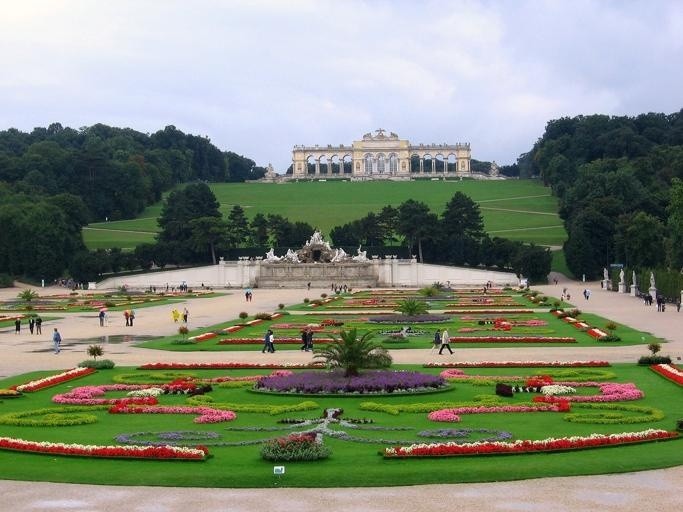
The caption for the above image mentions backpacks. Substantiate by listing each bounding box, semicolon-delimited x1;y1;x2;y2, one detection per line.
56;333;60;341
